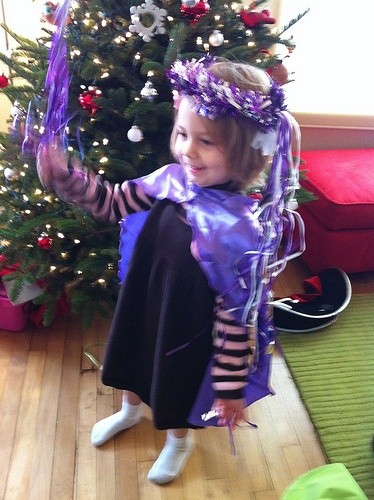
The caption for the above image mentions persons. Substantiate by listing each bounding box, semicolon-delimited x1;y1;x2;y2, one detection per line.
36;53;283;483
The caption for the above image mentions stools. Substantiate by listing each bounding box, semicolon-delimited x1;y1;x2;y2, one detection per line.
283;147;374;276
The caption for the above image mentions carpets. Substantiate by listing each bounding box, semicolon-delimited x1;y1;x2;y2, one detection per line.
271;292;374;500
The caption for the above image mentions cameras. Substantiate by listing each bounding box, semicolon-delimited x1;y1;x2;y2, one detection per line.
201;408;227;428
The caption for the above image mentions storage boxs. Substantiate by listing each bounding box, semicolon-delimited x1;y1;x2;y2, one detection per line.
0;284;31;332
